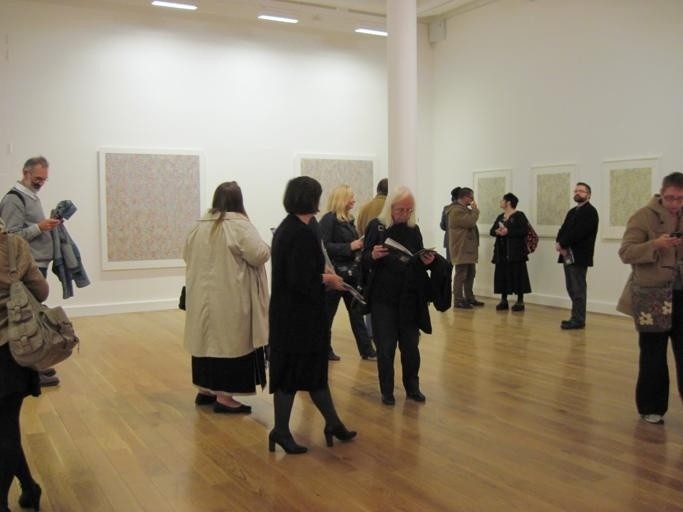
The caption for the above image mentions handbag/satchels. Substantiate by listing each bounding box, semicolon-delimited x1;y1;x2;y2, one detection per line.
521;223;539;255
632;285;674;332
6;280;79;372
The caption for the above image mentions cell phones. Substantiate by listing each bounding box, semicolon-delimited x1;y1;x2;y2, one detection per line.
670;232;683;238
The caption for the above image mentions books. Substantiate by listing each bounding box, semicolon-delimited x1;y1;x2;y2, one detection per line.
381;236;437;265
326;264;368;305
560;246;575;267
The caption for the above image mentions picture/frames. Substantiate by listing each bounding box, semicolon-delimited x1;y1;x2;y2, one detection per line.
474;170;510;234
601;155;661;238
295;156;375;216
531;163;575;240
99;147;203;272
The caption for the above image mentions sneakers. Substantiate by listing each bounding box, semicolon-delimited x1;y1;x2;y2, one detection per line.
640;414;664;424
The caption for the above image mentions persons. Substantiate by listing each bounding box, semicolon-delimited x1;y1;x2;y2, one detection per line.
490;192;538;312
0;231;50;511
553;181;599;330
181;181;271;415
444;186;486;309
614;171;681;425
440;186;462;269
1;155;64;388
319;183;379;361
263;174;357;453
359;178;420;339
358;184;439;405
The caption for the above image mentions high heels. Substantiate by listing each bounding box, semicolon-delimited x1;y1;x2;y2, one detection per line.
19;484;42;511
324;424;357;447
269;429;308;454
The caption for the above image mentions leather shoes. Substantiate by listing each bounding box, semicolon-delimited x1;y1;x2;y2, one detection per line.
561;320;585;329
382;391;426;405
454;300;524;311
195;393;251;413
40;368;59;386
327;350;377;360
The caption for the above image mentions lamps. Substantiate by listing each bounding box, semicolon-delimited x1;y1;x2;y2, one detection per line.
151;0;197;12
256;13;300;28
354;25;387;42
